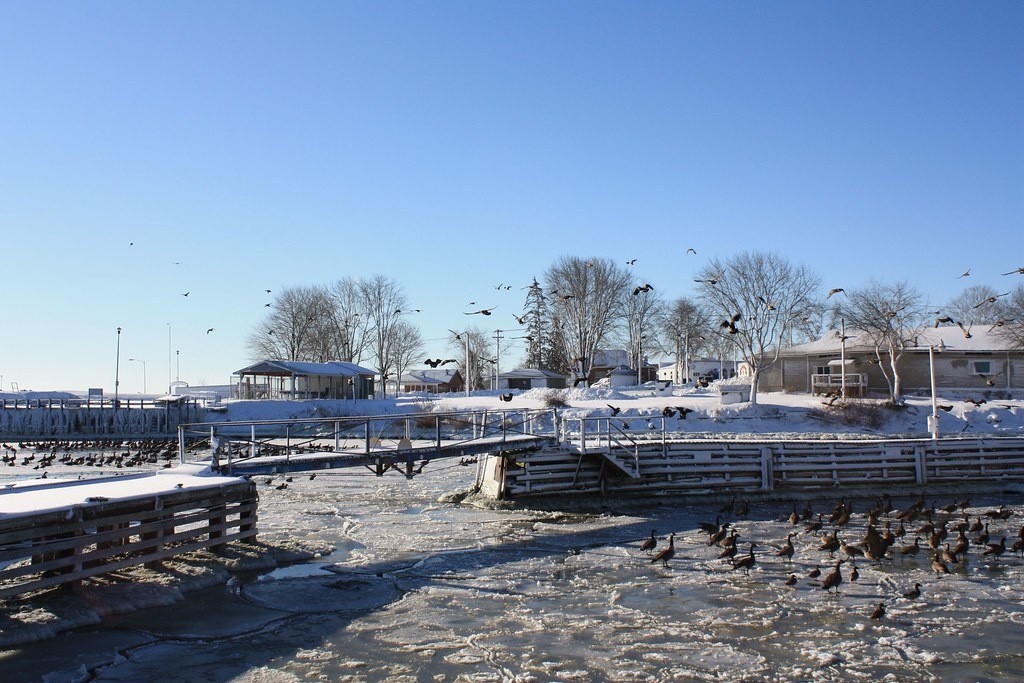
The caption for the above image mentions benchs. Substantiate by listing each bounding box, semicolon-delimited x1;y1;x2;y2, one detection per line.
279;390;326;400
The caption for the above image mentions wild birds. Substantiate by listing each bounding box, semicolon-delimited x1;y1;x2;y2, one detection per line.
220;437;359;493
422;355;499;369
639;489;1024;624
0;435;212;479
606;402;696;431
206;327;216;335
263;288;275;336
447;281;531;341
625;247;848;335
180;291;191;297
872;266;1024;412
